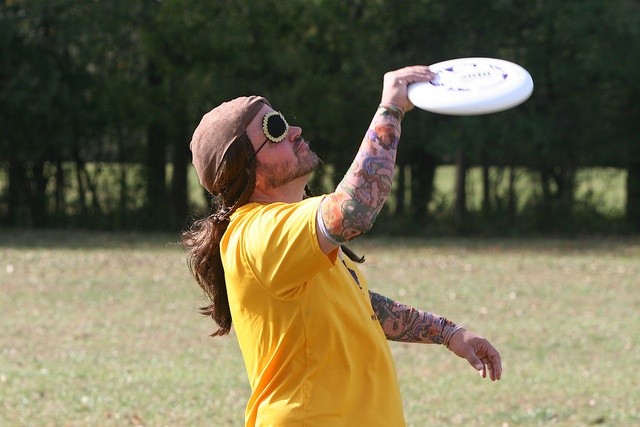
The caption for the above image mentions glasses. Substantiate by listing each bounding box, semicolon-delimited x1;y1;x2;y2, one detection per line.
254;107;292;155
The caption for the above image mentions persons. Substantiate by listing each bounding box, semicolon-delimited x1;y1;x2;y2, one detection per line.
181;64;503;427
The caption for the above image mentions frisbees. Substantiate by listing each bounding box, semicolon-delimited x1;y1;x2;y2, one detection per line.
406;56;534;116
